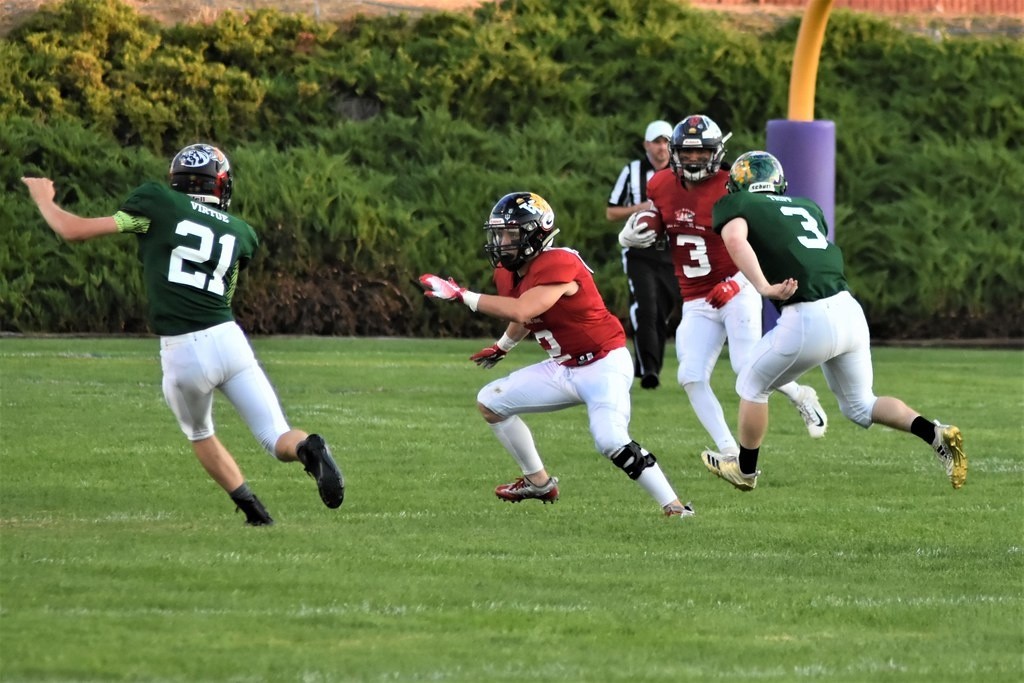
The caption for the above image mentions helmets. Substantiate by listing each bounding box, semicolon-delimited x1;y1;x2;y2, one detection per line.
483;191;560;272
725;150;788;195
169;143;233;212
668;115;728;184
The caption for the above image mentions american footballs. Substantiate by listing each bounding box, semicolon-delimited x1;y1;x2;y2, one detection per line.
632;210;664;242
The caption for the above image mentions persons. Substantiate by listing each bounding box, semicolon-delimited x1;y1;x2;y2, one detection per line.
419;191;696;519
20;144;344;526
700;149;968;491
607;121;684;389
618;114;828;456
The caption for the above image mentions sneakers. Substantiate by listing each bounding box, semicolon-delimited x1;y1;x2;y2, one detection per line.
495;475;559;504
701;445;761;492
665;501;696;518
795;386;828;439
932;419;967;489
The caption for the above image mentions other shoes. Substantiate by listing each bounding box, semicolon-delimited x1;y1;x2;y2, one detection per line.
640;371;659;390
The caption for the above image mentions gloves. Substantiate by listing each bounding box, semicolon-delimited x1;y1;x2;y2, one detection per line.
470;343;507;369
705;276;740;310
419;274;466;304
648;199;658;213
618;212;657;248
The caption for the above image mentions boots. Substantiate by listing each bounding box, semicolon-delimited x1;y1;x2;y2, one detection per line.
297;434;345;509
231;494;273;526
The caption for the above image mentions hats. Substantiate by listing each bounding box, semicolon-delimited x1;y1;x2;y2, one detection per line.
644;120;673;141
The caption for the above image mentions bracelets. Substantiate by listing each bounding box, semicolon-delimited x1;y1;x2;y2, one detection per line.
462;290;481;312
496;331;518;353
